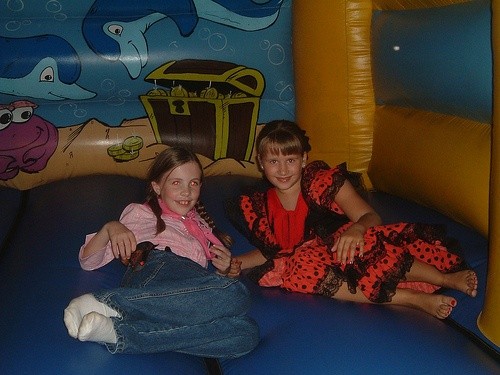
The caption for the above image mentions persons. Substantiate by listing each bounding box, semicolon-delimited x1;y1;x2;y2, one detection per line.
227;120;478;320
64;146;259;360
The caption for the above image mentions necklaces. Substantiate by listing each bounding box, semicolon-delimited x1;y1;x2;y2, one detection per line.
179;215;186;223
